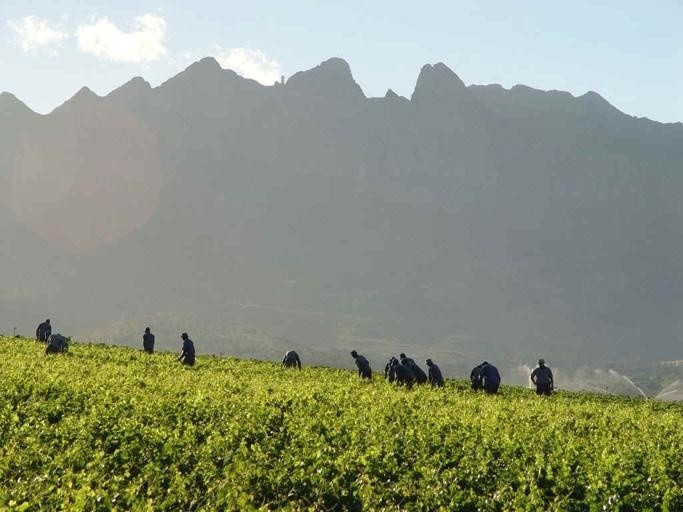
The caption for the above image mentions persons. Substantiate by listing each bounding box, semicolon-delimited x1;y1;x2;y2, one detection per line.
176;333;195;367
471;364;483;390
46;333;64;355
351;351;371;379
531;359;553;395
282;351;301;371
142;327;155;353
480;362;501;393
384;353;444;392
37;319;51;342
